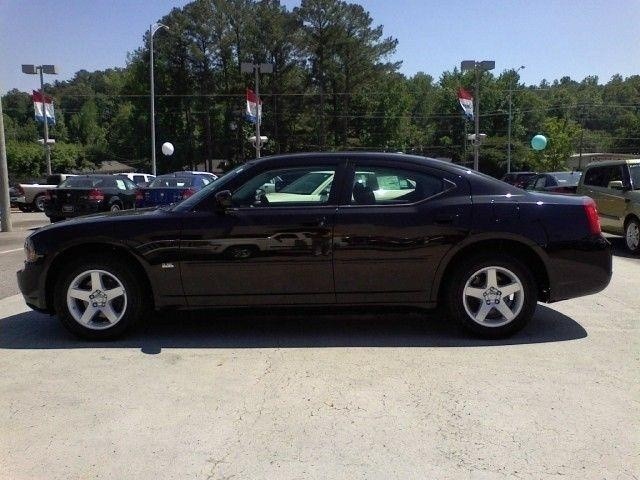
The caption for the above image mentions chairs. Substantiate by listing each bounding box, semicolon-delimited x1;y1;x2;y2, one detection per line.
353;182;376;203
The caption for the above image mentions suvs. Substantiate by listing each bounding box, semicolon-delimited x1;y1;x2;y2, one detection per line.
571;151;640;254
497;170;541;188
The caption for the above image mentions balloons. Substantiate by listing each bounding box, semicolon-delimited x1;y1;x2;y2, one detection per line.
532;135;547;151
161;141;175;156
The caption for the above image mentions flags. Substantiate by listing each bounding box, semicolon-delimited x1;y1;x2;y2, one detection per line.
244;88;262;127
33;90;56;126
459;87;474;119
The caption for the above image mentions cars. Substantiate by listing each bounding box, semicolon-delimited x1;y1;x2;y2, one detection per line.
260;169;415;203
8;171;222;221
16;154;616;340
530;168;582;193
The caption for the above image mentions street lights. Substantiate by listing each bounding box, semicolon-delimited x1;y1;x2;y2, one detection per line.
506;65;530;175
241;61;273;159
161;141;174;171
460;59;494;172
148;23;170;179
20;65;59;174
